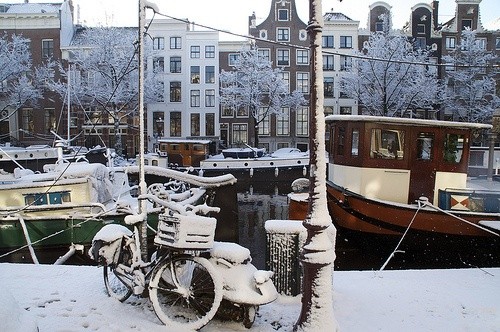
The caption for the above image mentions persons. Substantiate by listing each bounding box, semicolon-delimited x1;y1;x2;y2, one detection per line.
49;126;56;134
123;143;129;161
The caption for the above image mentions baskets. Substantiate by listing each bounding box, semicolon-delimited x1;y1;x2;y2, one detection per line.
154;214;216;249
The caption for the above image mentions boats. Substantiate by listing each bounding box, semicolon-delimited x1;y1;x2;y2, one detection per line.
324;115;500;241
0;138;329;249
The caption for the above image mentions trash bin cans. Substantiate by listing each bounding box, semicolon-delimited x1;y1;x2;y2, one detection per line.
264;219;307;296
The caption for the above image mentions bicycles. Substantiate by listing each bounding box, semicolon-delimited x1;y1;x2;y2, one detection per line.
98;187;223;332
